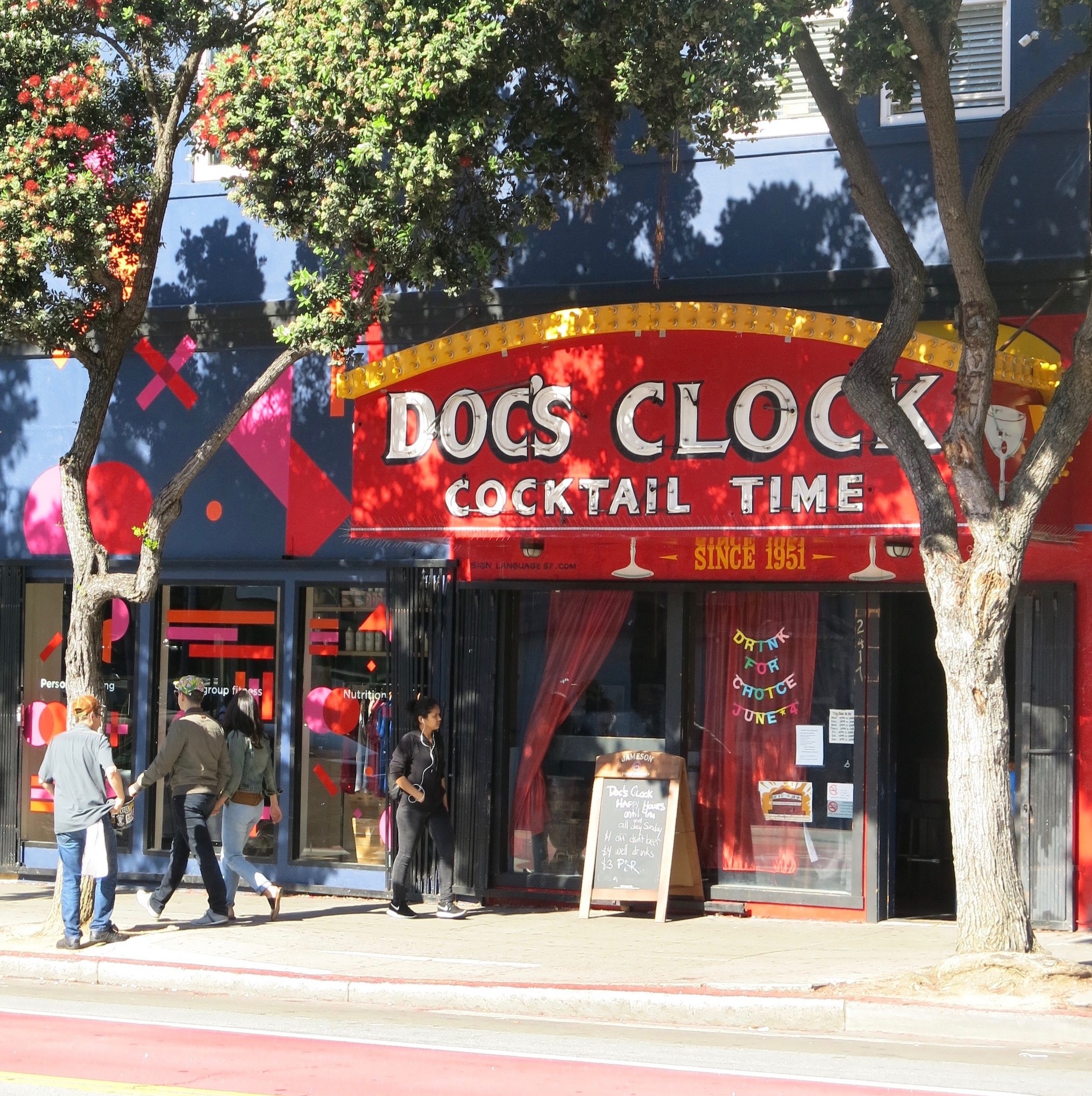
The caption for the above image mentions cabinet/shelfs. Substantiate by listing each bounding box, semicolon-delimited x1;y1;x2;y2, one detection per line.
315;603;427;657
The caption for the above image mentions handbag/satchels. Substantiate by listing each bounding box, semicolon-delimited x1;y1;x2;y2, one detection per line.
231;791;264;806
387;732;414;800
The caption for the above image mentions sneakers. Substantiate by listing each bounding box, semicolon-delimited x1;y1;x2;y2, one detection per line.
436;901;468;919
136;889;161;918
387;900;417;918
191;908;228;926
89;920;129;942
56;936;80;949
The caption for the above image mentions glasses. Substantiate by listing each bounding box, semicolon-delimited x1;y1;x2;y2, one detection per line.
174;689;184;698
95;711;104;721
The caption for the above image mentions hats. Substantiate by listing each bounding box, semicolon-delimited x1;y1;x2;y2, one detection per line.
173;675;204;698
71;696;106;718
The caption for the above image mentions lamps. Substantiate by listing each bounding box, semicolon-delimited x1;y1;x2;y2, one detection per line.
520;542;544;557
885;541;914;557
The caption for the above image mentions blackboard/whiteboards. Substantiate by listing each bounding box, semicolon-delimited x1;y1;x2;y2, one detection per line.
582;749;704;900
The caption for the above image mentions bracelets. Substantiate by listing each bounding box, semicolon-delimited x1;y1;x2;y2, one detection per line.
212;810;219;814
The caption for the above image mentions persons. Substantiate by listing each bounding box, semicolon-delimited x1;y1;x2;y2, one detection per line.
37;695;130;949
1008;755;1016;819
211;690;284;922
128;675;231;927
386;699;468;919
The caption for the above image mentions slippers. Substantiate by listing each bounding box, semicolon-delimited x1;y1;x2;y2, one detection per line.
268;887;283;921
227;915;236;921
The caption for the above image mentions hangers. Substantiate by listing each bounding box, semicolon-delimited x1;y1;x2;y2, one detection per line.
360;682;392;705
